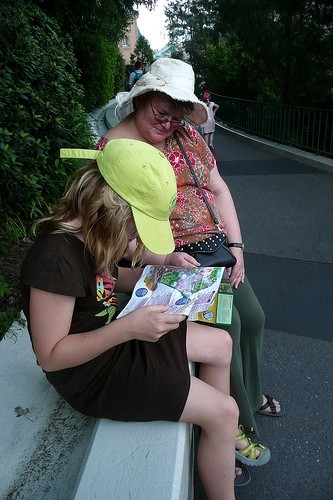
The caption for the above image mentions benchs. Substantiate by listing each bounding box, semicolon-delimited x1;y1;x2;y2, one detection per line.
0;308;196;500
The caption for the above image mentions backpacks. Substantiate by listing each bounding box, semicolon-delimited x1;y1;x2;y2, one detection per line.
133;70;143;84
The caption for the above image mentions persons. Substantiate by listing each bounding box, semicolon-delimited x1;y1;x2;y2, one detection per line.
199;92;220;148
128;62;145;87
98;59;282;486
23;138;271;500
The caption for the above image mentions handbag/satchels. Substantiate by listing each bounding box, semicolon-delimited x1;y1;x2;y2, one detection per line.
176;233;238;269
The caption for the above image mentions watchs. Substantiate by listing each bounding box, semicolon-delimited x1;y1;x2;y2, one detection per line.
224;241;244;249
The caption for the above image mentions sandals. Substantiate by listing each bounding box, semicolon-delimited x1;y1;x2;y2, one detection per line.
235;423;272;466
234;458;252;486
257;394;286;416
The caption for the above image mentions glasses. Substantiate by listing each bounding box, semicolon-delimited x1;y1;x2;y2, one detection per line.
149;101;186;129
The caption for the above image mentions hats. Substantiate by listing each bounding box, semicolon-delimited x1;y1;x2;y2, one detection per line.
60;138;178;256
115;57;210;126
201;91;212;102
